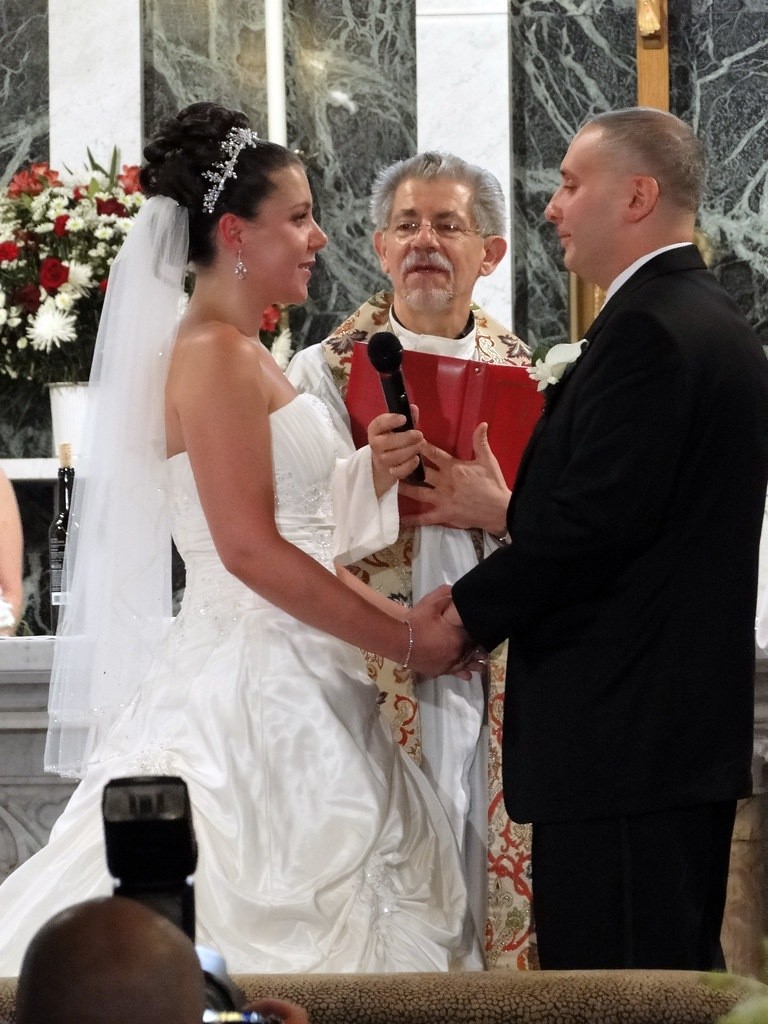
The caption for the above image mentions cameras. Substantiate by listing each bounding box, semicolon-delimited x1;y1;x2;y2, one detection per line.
102;775;273;1024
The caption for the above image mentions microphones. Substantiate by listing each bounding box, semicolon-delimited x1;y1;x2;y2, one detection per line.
366;331;426;484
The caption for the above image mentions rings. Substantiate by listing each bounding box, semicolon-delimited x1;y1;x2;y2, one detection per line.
460;652;467;664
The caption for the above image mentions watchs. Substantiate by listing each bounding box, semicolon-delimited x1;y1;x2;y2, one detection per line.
499;530;512;546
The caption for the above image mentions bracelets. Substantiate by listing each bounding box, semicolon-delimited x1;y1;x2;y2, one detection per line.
402;619;413;669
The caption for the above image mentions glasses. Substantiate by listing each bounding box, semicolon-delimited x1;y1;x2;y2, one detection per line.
382;217;495;244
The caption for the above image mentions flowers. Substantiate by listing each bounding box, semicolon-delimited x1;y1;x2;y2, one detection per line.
0;146;146;421
526;338;587;392
259;304;292;372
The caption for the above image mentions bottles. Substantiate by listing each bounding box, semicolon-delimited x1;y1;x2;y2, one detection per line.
48;466;76;636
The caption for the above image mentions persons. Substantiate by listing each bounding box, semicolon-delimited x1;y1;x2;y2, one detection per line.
0;100;768;1024
